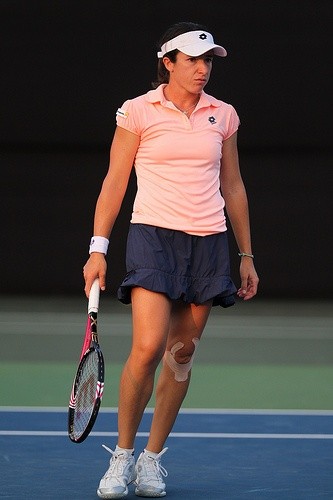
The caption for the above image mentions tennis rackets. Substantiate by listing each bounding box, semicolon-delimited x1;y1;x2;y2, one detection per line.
68;278;105;442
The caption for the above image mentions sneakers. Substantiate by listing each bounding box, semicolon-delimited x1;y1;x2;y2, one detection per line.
135;447;171;495
96;443;138;498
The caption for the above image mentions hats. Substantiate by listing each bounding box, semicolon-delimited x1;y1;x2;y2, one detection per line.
157;30;228;59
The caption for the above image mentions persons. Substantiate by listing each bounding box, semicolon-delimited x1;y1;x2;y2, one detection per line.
83;23;259;498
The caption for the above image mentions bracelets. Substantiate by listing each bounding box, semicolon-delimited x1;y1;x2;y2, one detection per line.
238;253;254;258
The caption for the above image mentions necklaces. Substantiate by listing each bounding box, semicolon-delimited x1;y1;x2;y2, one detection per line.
168;97;197;115
